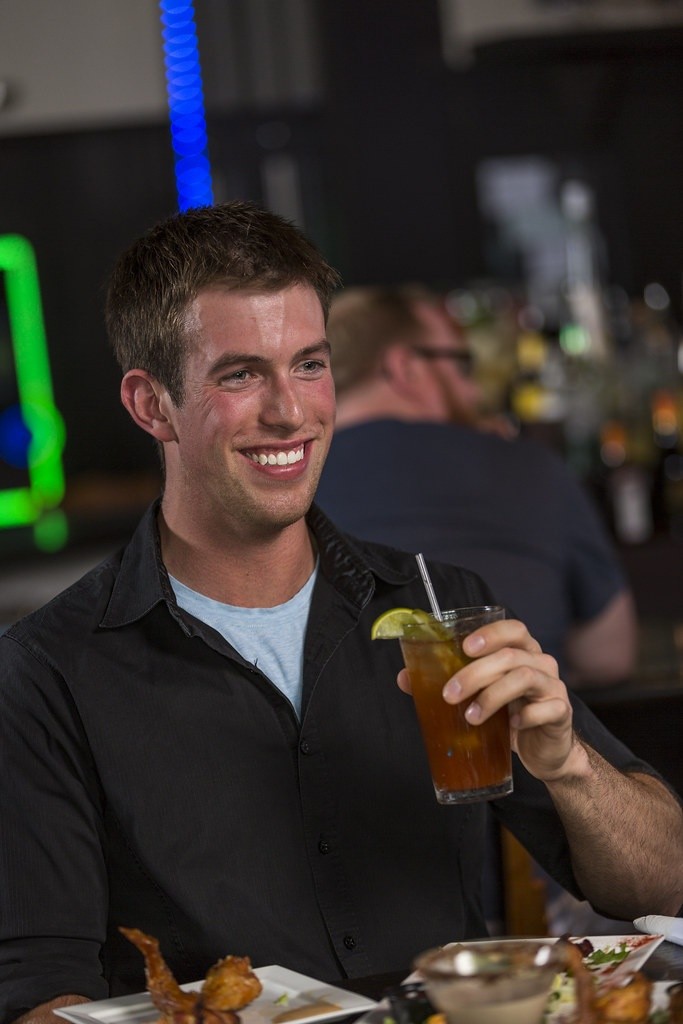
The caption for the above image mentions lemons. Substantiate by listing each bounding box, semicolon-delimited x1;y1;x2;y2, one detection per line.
370;607;420;639
412;608;462;672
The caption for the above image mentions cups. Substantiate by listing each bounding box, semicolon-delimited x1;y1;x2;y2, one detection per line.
399;605;513;804
416;939;563;1024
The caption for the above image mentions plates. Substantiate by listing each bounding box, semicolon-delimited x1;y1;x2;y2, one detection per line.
356;934;667;1024
52;965;380;1024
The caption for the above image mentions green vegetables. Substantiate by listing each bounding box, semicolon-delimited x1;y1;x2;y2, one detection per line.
469;945;629;1017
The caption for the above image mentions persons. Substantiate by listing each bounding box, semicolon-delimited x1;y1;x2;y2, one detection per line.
315;286;634;686
0;205;683;1023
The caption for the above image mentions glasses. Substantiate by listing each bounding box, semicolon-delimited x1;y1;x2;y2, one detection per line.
410;341;475;380
